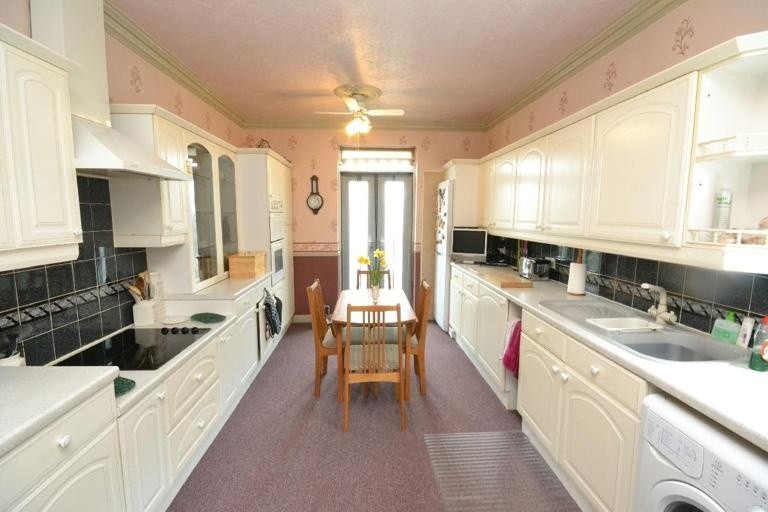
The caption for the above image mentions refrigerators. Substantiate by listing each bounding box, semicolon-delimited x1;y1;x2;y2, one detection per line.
433;180;453;333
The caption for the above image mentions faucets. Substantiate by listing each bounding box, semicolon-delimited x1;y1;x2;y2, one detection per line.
640;282;668;328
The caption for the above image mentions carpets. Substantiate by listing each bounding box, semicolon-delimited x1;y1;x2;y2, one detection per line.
421;428;582;511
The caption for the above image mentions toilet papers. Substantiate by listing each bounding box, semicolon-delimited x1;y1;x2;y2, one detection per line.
566;262;587;295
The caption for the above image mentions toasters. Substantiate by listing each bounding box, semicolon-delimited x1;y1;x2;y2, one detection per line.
518;256;551;281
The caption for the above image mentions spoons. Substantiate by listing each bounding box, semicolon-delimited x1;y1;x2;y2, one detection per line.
127;269;150;301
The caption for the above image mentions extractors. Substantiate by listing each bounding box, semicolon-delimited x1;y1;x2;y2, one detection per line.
70;114;195;182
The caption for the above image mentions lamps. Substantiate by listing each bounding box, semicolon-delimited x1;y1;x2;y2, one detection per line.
342;116;372;137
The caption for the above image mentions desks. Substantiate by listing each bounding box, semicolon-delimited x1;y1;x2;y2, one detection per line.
331;286;419;403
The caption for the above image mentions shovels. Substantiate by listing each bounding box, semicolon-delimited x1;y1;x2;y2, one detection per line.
136;277;145;300
128;285;143;301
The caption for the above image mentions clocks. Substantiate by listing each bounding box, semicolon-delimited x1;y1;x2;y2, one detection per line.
306;176;323;215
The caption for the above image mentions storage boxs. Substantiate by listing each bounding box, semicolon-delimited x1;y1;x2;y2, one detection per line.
228;249;266;280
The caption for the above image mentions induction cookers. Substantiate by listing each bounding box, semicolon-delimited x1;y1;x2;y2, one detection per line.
50;328;211;370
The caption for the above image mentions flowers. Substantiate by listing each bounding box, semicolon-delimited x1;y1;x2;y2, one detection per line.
355;248;392;287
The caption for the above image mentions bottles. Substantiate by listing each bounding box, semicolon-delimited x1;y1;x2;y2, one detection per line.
713;178;733;228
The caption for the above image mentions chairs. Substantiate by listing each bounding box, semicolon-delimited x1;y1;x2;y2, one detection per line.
356;269;391;290
373;278;432;401
306;277;369;400
345;304;405;430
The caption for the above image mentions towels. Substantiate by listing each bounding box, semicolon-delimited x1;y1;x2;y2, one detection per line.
262;287;284;341
501;318;520;360
502;320;521;377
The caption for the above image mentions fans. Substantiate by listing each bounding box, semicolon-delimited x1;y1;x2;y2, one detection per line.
314;85;404;116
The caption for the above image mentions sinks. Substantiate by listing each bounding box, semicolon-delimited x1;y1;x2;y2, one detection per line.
584;316;665;332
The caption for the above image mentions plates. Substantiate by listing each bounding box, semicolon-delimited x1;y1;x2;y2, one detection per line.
162;317;186;324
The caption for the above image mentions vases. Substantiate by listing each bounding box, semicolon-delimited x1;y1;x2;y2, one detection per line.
368;284;380;305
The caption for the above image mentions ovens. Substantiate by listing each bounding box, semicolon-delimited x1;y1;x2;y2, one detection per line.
270;238;287;286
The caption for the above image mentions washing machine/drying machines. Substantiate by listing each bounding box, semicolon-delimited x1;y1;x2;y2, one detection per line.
631;392;768;511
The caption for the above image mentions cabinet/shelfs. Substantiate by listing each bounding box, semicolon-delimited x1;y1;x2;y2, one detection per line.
138;109;237;298
274;271;297;342
679;28;767;275
514;101;594;248
229;273;272;394
518;306;649;511
476;274;522;409
117;381;169;512
106;102;186;249
448;263;479;362
0;21;85;274
479;143;514;238
233;146;296;243
443;156;479;226
168;330;227;505
0;364;130;512
589;66;699;249
